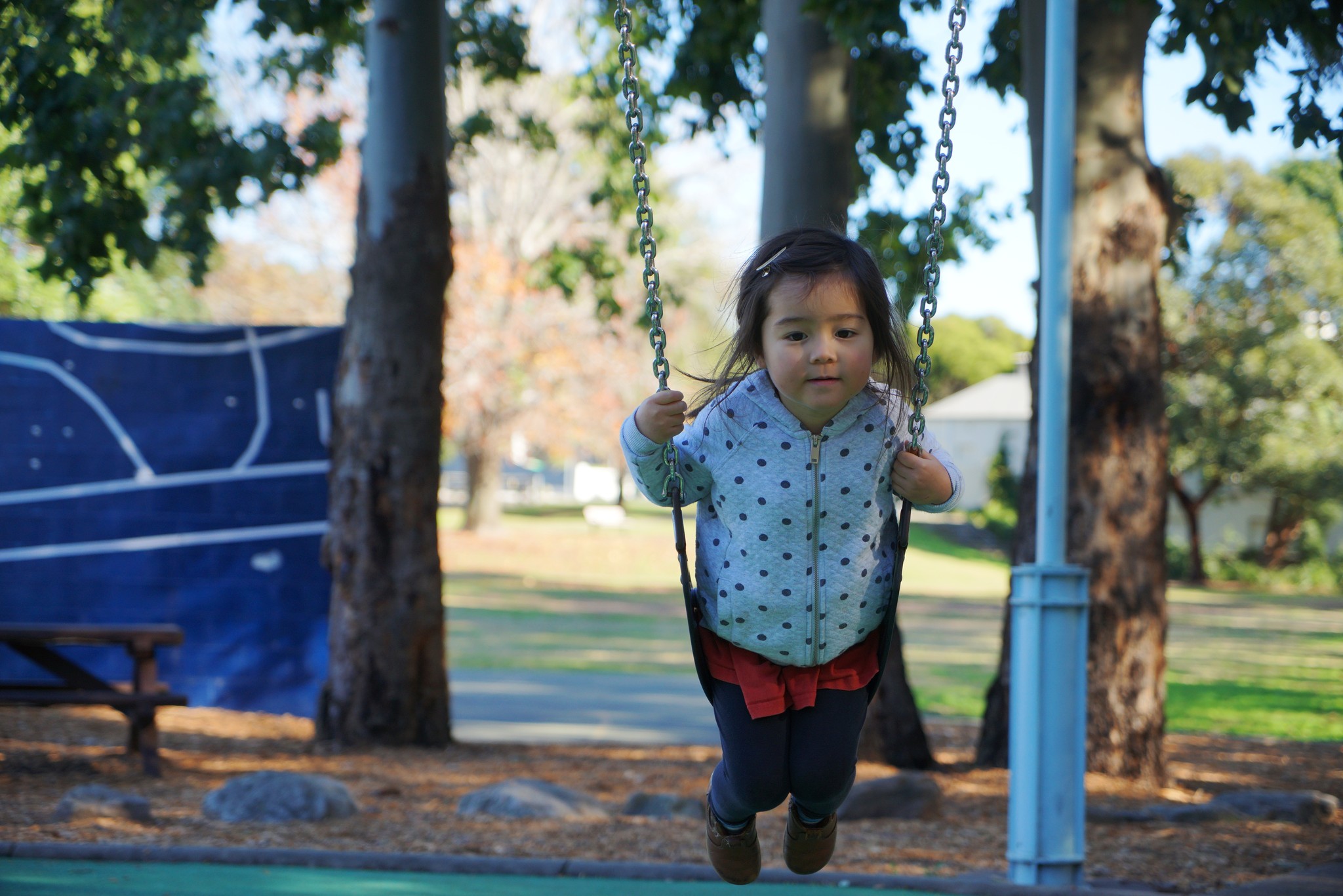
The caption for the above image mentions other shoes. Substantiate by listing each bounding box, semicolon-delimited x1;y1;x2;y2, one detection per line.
783;794;838;875
706;791;761;885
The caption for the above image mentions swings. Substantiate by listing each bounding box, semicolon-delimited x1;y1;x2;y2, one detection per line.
606;0;964;699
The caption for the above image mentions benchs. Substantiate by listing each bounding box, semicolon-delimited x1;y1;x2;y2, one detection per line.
0;677;189;710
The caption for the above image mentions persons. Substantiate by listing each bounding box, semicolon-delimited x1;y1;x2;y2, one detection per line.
618;228;961;885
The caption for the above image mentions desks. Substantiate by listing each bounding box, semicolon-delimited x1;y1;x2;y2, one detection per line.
0;620;184;781
